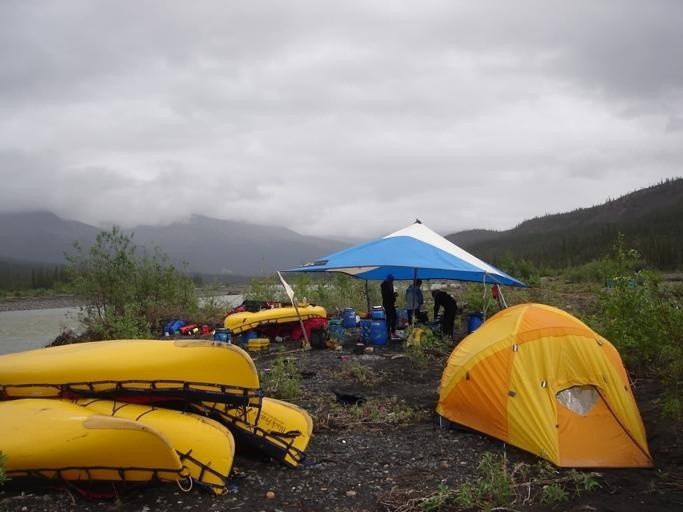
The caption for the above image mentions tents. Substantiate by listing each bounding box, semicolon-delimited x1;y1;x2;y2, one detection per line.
435;303;656;468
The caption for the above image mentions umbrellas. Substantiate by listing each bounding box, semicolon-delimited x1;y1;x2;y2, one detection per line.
280;219;533;329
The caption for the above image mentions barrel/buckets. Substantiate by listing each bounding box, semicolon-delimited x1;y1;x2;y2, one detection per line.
214;328;232;344
467;312;482;331
343;306;408;345
243;331;257;343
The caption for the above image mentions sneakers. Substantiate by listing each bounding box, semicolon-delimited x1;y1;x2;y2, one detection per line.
390;334;401;340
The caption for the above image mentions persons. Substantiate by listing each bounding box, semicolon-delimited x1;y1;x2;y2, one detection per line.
380;274;401;339
432;289;457;339
405;278;423;326
